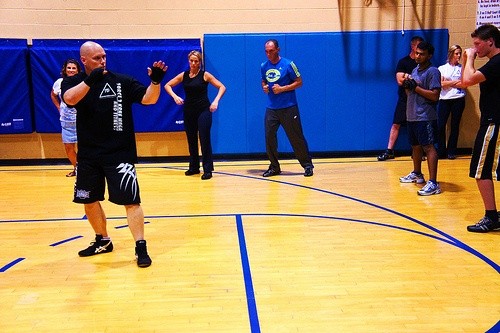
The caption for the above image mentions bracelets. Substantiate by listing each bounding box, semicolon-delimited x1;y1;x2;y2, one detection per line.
152;82;159;85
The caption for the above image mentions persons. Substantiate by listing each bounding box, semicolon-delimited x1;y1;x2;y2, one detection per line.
260;39;314;177
461;24;500;232
438;45;466;159
378;36;425;159
51;59;82;177
164;50;226;179
61;41;168;266
399;42;441;196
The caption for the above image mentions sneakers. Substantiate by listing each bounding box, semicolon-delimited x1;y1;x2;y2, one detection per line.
399;173;424;183
202;171;212;179
135;240;151;267
79;234;113;256
417;182;440;195
185;169;200;175
468;211;500;232
378;151;395;161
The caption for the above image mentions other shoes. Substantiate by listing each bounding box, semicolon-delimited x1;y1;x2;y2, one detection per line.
263;168;278;177
304;168;312;175
448;153;455;159
66;171;75;177
